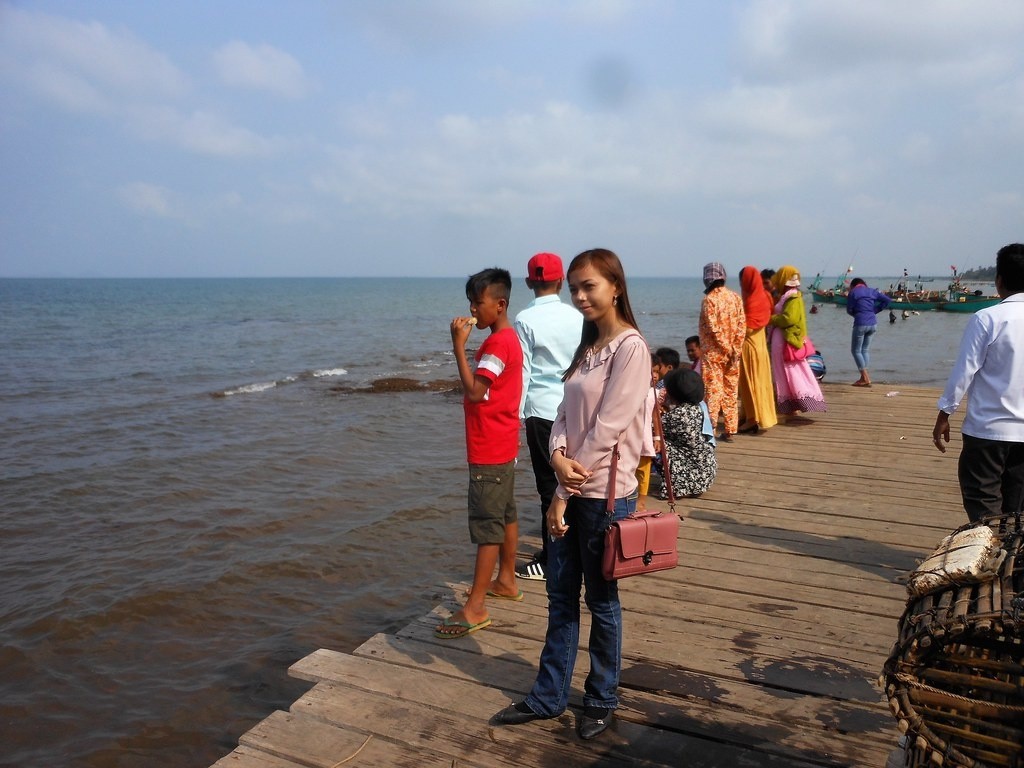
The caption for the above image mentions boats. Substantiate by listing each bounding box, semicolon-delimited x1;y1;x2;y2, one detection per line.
808;265;1003;312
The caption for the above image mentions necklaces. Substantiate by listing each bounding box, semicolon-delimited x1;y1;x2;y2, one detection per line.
590;322;625;355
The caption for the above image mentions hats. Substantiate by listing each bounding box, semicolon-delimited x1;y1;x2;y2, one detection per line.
785;273;800;287
528;252;564;282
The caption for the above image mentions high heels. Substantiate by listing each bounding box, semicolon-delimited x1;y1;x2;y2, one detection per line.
738;424;758;435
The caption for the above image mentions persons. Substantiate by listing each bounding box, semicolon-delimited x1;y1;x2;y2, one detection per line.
846;277;890;388
698;262;746;444
738;266;777;433
632;354;662;517
684;336;702;373
761;268;779;302
767;267;827;423
651;368;718;499
655;347;680;375
932;243;1024;524
512;254;586;580
430;266;525;639
492;250;651;740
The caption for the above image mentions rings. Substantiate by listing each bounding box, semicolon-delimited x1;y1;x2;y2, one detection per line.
551;526;556;529
933;440;936;443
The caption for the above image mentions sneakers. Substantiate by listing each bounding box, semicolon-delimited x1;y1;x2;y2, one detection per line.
515;561;547;581
533;549;544;561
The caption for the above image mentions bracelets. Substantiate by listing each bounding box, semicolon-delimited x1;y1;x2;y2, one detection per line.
653;436;660;441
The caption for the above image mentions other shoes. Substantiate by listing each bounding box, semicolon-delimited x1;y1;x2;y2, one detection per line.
492;701;544;725
719;434;733;443
579;705;614;740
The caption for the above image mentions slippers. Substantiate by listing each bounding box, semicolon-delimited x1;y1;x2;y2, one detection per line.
432;616;493;638
487;584;524;602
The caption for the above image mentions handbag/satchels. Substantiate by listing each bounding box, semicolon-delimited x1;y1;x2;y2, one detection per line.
784;336;816;363
602;510;684;582
